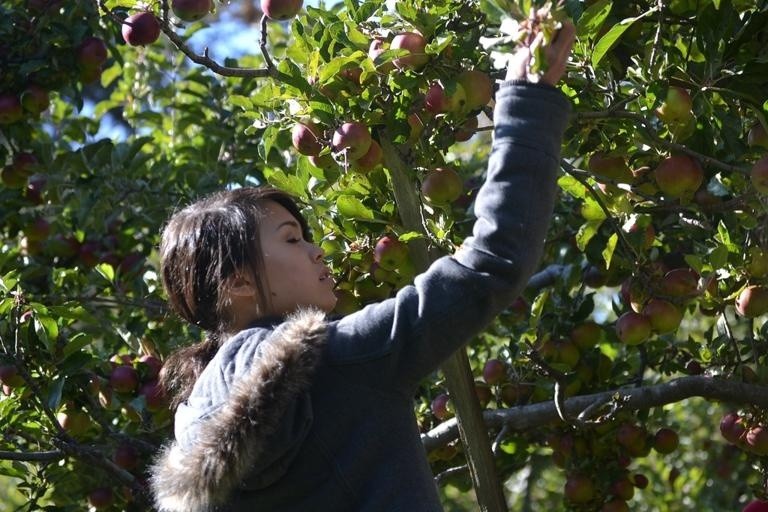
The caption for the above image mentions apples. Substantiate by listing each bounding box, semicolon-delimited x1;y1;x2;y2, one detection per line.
121;12;161;47
0;0;107;125
260;0;304;21
172;0;212;22
0;354;172;508
291;32;768;512
0;152;145;293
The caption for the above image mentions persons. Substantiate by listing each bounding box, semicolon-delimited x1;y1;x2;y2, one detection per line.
143;8;579;512
